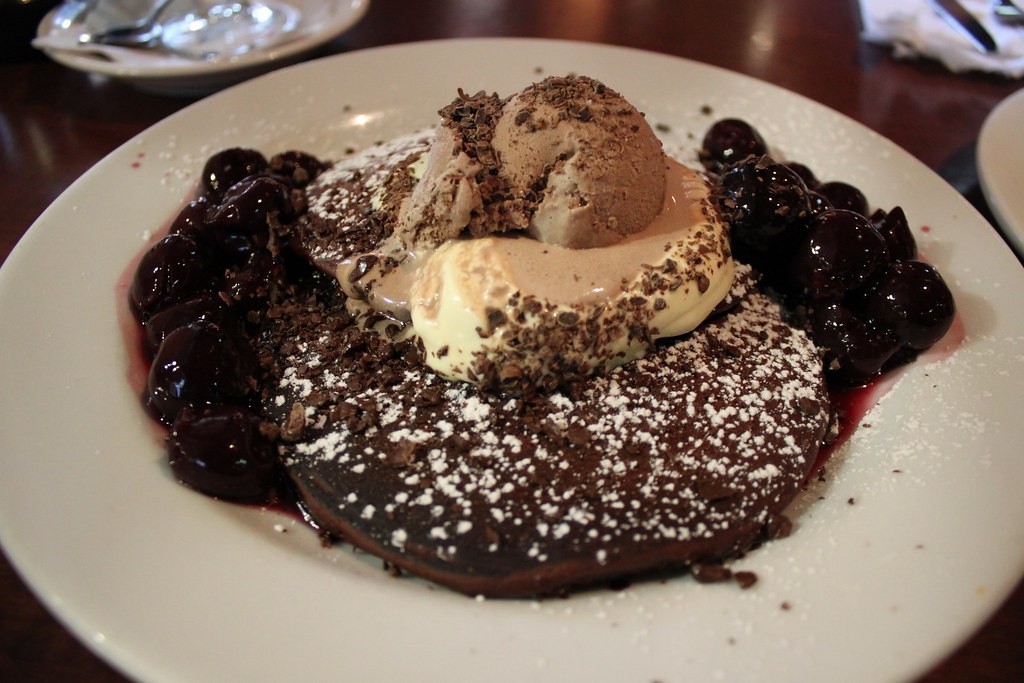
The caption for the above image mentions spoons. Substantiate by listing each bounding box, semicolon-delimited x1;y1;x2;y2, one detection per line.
79;0;175;46
993;0;1024;29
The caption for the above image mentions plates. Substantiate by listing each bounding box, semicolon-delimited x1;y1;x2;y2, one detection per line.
977;89;1024;252
0;39;1024;683
39;0;370;81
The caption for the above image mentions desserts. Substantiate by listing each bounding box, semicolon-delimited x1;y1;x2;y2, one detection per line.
266;70;832;600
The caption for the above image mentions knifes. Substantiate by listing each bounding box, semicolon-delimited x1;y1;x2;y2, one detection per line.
925;0;998;55
